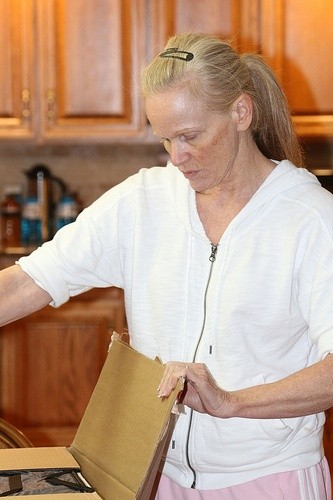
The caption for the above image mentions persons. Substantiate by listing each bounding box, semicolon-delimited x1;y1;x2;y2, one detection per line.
0;31;332;500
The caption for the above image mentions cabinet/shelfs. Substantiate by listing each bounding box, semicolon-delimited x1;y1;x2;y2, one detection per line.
0;0;333;147
0;287;129;447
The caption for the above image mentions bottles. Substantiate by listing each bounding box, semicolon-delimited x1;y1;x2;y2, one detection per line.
1;194;21;247
55;196;77;231
21;197;42;246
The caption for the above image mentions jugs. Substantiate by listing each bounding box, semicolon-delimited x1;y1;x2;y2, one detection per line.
23;164;66;241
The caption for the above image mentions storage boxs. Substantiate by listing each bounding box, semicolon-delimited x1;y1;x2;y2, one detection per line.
0;331;188;500
